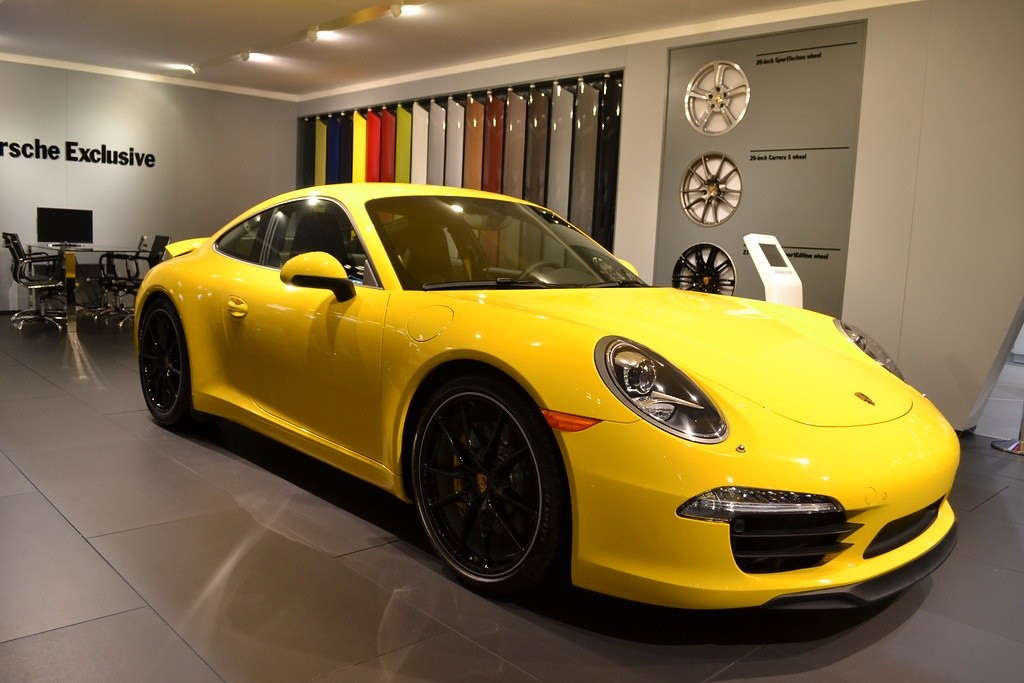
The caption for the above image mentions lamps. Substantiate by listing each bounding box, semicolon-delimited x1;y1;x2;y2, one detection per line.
390;0;403;17
190;64;200;74
306;25;319;41
239;47;252;60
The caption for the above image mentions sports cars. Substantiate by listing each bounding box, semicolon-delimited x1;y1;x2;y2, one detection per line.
134;182;962;613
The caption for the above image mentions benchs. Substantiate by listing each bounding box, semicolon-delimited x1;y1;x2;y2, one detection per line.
276;253;367;278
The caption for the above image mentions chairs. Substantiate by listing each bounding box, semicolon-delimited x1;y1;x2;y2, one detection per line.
0;232;68;333
290;214;351;276
404;224;463;283
91;236;170;328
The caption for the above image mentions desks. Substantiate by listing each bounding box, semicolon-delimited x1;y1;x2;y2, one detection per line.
26;242;164;332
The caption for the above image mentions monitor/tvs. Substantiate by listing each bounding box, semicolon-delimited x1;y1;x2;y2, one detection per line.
37;207;93;247
